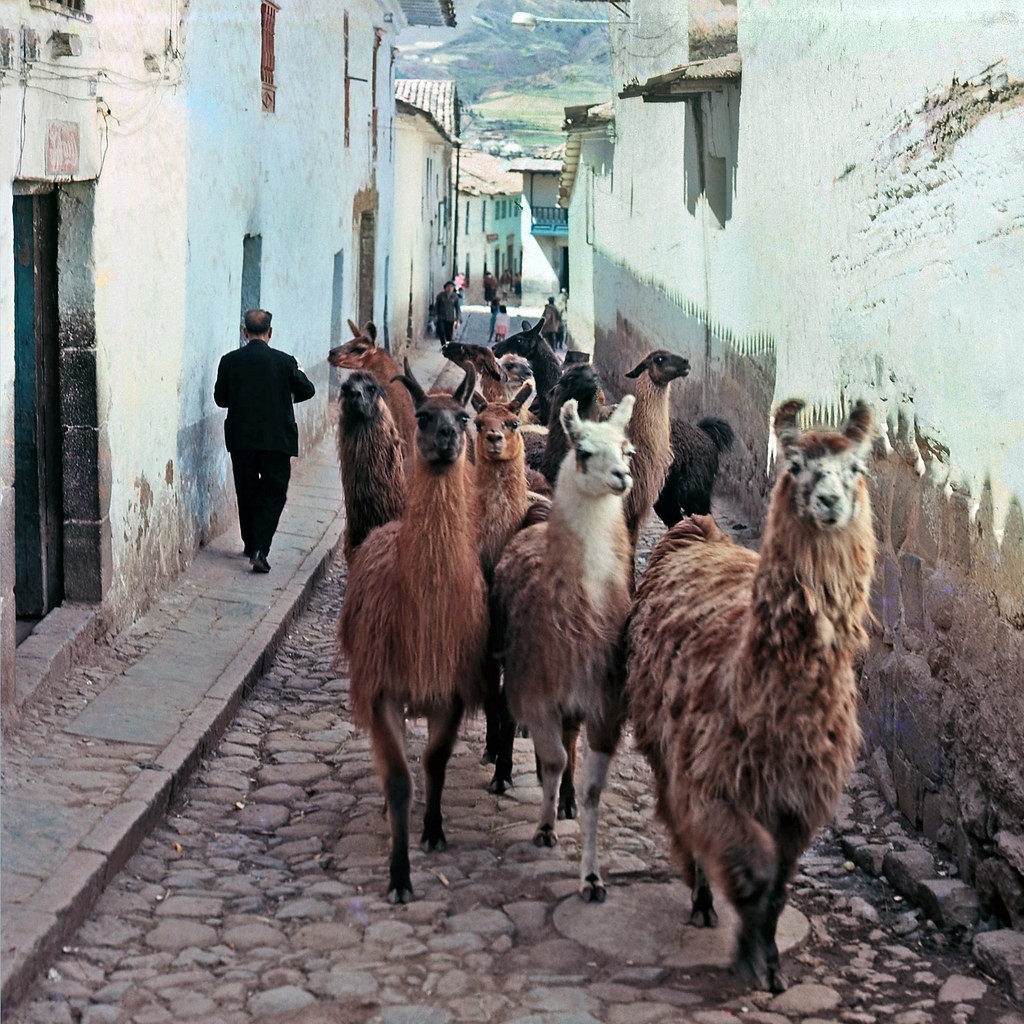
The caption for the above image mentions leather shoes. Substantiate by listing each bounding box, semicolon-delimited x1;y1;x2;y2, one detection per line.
250;550;272;572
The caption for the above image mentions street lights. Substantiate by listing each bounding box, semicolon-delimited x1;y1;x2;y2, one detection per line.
508;11;606;32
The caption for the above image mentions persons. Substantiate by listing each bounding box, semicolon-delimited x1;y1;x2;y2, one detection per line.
488;297;499;341
495;305;510;342
542;296;564;353
500;269;512;301
214;309;316;574
555;288;569;317
484;273;497;305
512;272;521;298
434;281;463;349
